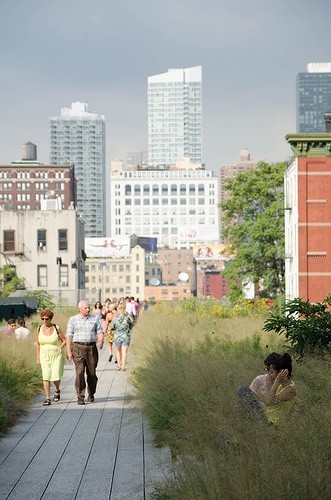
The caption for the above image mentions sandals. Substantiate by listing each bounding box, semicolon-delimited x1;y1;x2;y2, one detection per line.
53;390;60;402
43;399;51;406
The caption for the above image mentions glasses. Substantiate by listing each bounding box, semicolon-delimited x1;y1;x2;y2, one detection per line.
264;367;269;371
41;317;50;321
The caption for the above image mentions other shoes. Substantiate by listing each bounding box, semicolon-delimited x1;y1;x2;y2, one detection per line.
108;354;113;362
88;394;94;402
78;398;85;405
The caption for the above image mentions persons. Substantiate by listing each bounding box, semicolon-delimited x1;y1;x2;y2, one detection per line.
236;353;298;429
1;319;17;335
249;352;283;404
15;319;31;340
104;304;117;364
91;296;149;331
109;303;133;371
65;300;104;405
36;310;67;405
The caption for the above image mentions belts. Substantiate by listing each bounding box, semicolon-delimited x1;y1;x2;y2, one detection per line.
74;342;96;346
